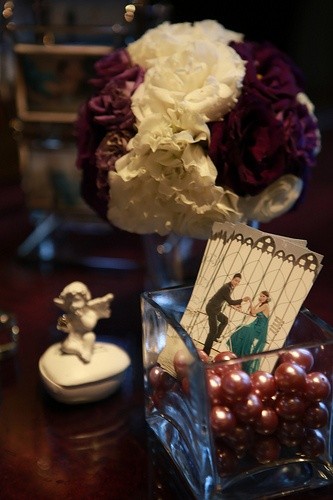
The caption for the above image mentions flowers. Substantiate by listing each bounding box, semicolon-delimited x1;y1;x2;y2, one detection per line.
74;19;321;242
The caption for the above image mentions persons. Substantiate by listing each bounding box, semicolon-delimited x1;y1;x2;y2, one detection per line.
204;274;247;358
226;290;272;376
52;283;113;364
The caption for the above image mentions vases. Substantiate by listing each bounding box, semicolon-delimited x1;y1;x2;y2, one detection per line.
135;217;261;297
